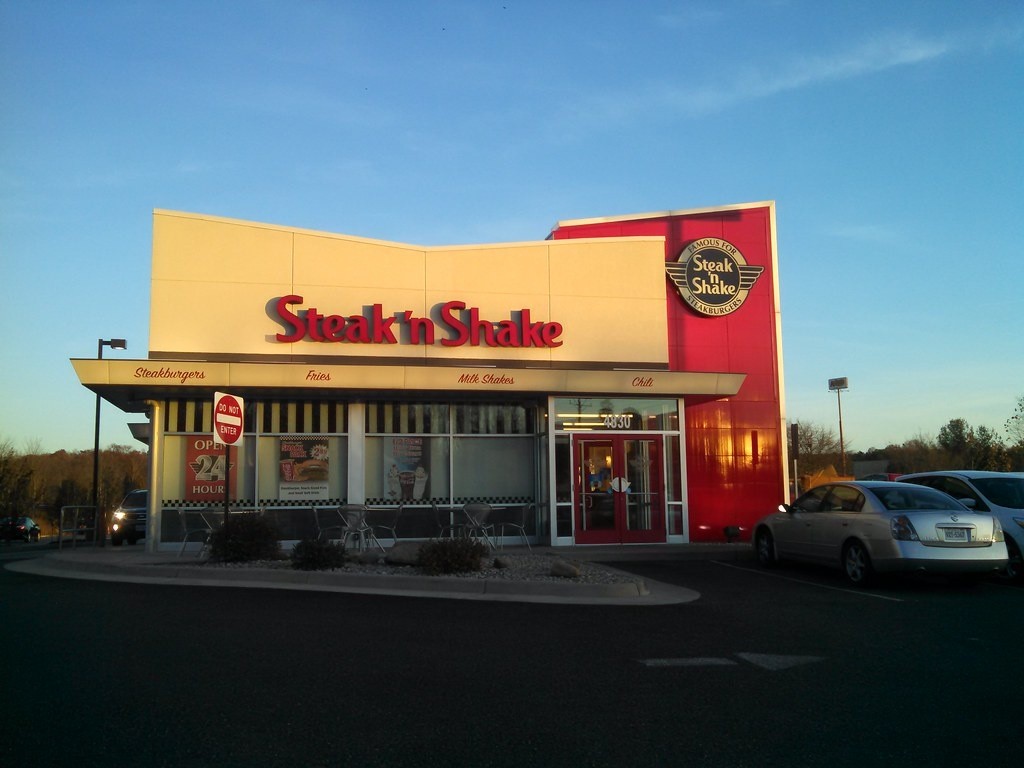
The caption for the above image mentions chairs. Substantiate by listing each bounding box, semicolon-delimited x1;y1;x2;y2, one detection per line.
309;500;403;553
176;506;266;559
432;499;538;552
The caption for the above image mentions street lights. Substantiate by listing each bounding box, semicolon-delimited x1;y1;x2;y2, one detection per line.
92;339;126;509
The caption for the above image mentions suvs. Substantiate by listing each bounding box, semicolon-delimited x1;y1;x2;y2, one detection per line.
109;489;147;545
894;470;1024;583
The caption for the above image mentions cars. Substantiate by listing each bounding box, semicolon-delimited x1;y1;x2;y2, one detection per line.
0;517;41;543
753;481;1009;588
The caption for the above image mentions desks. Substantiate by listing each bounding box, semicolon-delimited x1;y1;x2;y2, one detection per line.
334;508;398;548
438;506;507;544
206;511;259;526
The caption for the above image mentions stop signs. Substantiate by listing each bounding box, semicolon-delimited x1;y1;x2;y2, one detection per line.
213;391;244;447
